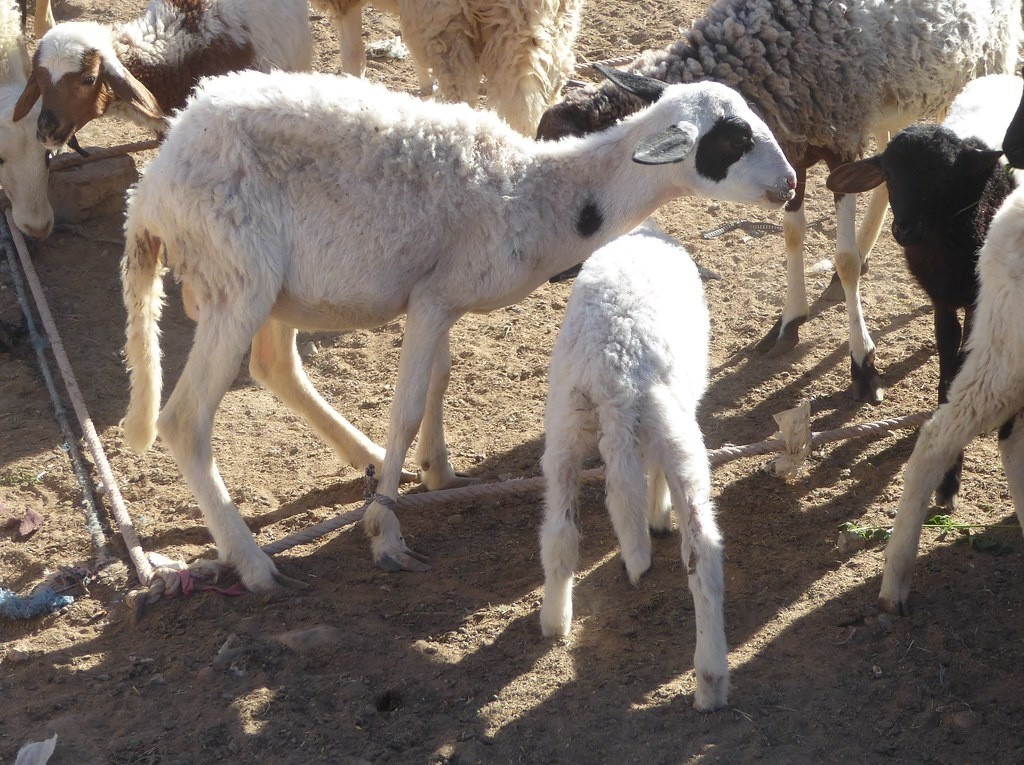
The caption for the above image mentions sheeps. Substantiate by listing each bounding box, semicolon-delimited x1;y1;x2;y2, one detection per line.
13;0;315;148
317;0;582;139
540;214;734;712
120;78;798;601
876;185;1024;613
826;75;1024;441
0;0;59;239
537;0;1024;397
